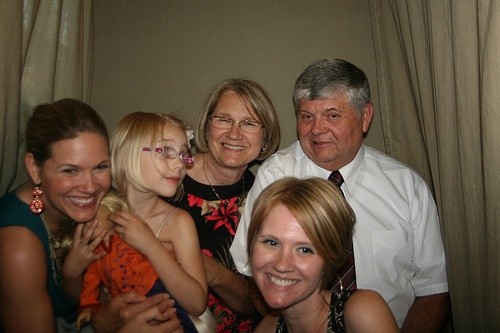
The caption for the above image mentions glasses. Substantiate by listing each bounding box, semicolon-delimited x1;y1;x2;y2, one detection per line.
210;115;265;133
143;146;194;164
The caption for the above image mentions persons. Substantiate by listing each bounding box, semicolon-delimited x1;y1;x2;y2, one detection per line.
61;109;218;333
229;55;450;333
75;193;198;333
245;175;398;332
1;98;181;333
81;75;280;333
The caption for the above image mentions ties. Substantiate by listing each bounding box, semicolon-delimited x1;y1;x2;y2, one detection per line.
327;170;357;291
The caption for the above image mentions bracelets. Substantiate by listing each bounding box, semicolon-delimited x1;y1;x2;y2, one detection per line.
79;321;96;332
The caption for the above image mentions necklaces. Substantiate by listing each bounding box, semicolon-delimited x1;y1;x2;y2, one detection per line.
41;212;64;287
202;152;244;205
